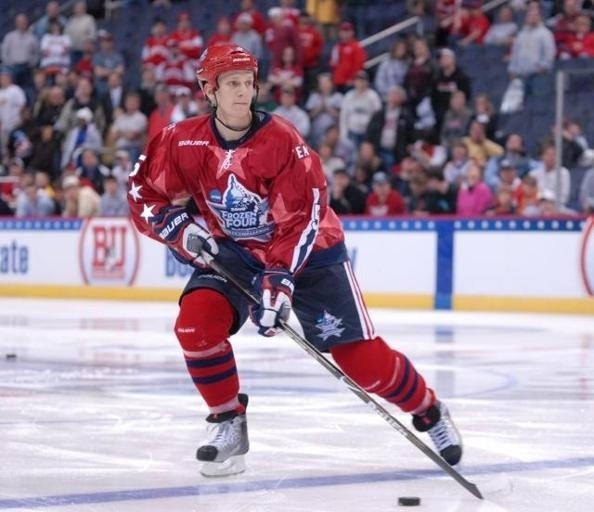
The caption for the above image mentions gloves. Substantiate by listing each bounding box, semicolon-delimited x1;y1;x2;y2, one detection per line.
246;268;297;338
148;204;220;270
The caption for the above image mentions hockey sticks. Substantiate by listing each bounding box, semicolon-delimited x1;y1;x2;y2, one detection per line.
200;248;538;501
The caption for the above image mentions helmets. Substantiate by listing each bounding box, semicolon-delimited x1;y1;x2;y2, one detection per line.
195;40;259;101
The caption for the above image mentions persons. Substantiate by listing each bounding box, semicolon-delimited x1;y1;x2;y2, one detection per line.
126;41;462;465
0;1;265;218
263;2;594;218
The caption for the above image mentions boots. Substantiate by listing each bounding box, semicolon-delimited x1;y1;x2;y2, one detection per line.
412;400;463;466
197;393;250;462
